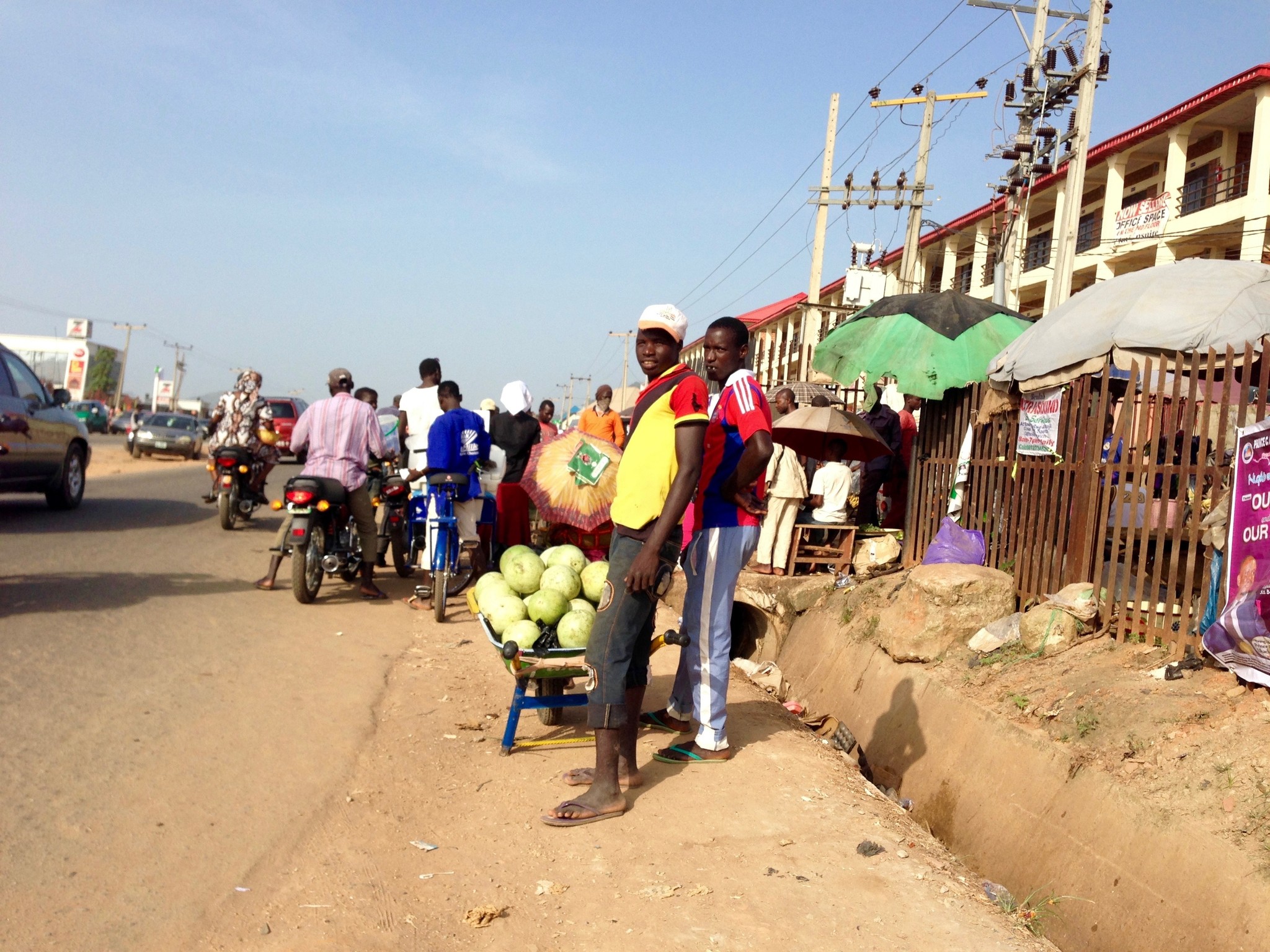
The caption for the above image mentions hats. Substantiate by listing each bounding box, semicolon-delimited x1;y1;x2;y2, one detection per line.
638;303;688;343
480;398;496;411
325;368;352;387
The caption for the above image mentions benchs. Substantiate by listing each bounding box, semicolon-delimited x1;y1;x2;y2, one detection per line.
788;523;859;577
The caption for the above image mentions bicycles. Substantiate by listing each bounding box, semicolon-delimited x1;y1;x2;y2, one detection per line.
402;448;497;623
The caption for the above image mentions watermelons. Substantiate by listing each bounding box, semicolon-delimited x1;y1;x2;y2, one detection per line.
475;544;610;649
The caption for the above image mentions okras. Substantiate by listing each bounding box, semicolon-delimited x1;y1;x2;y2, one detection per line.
856;523;883;532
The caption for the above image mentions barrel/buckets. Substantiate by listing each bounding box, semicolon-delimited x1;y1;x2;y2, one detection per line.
378;414;401;455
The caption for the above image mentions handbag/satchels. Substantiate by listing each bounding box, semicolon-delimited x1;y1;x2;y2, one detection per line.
253;423;279;444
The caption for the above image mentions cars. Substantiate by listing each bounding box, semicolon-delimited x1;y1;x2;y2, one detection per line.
0;342;93;512
263;395;309;465
109;408;219;461
64;400;108;434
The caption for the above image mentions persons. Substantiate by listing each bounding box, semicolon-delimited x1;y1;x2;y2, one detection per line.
638;316;774;766
203;358;559;610
578;384;625;447
538;303;708;827
1041;406;1150;567
1203;555;1270;660
750;379;923;577
108;403;200;455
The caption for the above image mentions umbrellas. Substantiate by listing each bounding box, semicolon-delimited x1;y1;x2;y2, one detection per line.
521;427;624;532
764;255;1270;410
769;405;893;461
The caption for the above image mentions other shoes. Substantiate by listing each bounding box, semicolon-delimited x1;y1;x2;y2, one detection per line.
202;493;219;503
251;487;270;505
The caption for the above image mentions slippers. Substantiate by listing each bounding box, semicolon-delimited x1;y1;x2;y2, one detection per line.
562;768;642;789
741;564;757;574
401;595;420;610
540;801;623;826
652;744;727;764
639;712;692;735
360;587;389;600
253;578;272;591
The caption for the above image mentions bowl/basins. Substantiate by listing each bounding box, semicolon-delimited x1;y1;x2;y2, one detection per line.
399;468;416;480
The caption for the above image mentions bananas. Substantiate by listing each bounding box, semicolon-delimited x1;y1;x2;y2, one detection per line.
847;495;859;509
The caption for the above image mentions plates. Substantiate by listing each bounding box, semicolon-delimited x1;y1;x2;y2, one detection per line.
855;528;903;536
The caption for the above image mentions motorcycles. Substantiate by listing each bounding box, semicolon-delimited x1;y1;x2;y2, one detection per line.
283;474;427;605
201;444;269;530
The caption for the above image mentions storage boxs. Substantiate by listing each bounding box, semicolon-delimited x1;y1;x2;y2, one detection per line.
852;533;900;575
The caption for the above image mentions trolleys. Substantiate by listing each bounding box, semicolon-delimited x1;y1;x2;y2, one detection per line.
464;585;693;757
